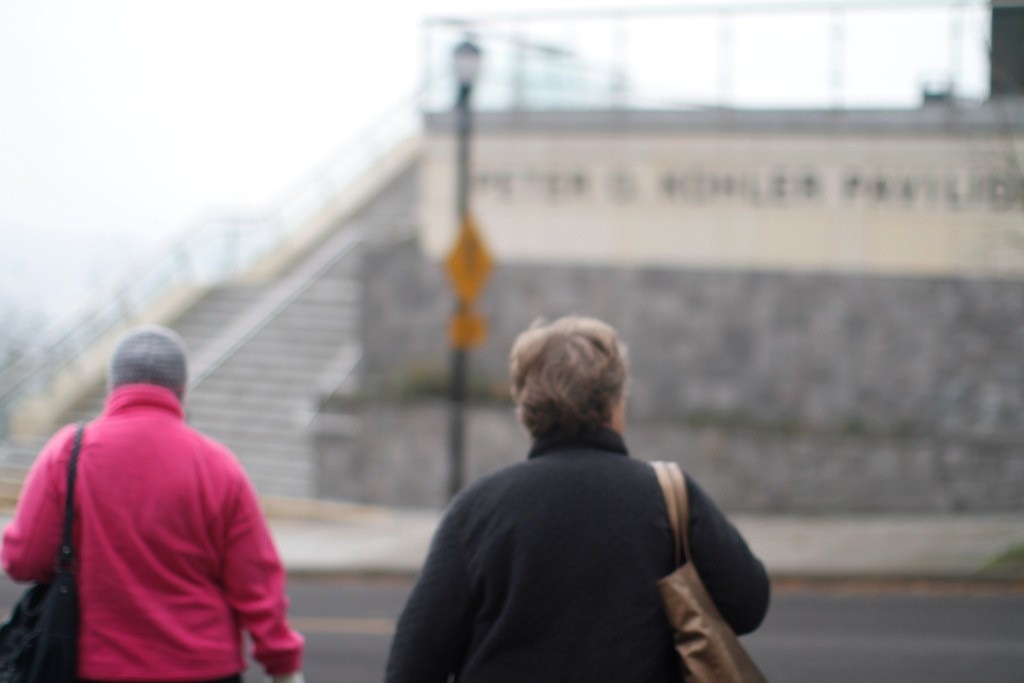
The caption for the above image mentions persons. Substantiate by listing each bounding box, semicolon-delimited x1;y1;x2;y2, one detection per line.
385;318;768;683
0;325;305;683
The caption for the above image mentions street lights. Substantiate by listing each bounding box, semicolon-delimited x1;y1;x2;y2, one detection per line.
446;40;486;502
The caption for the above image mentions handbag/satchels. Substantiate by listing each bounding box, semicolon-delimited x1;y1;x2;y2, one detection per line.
0;569;78;683
648;461;769;683
